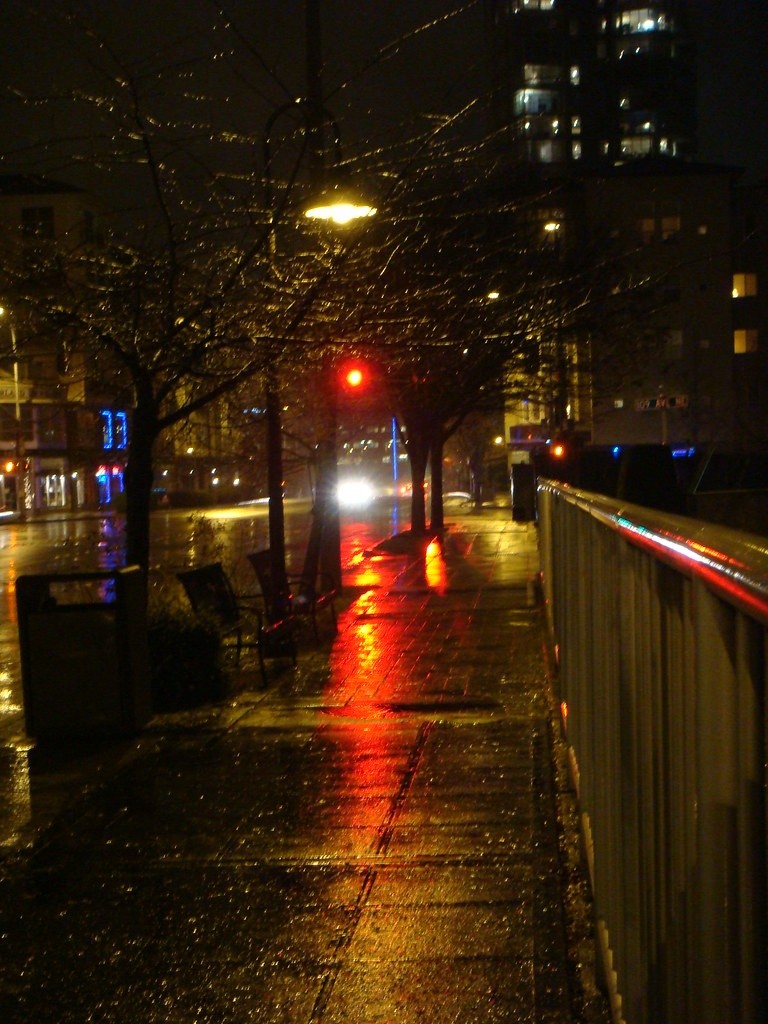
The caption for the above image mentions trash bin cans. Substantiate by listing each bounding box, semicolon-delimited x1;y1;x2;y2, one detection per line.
12;563;150;746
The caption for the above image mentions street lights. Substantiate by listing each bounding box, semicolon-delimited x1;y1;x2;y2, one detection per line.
260;99;382;574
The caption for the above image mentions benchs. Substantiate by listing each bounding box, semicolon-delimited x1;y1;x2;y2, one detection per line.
179;562;299;688
247;545;340;642
